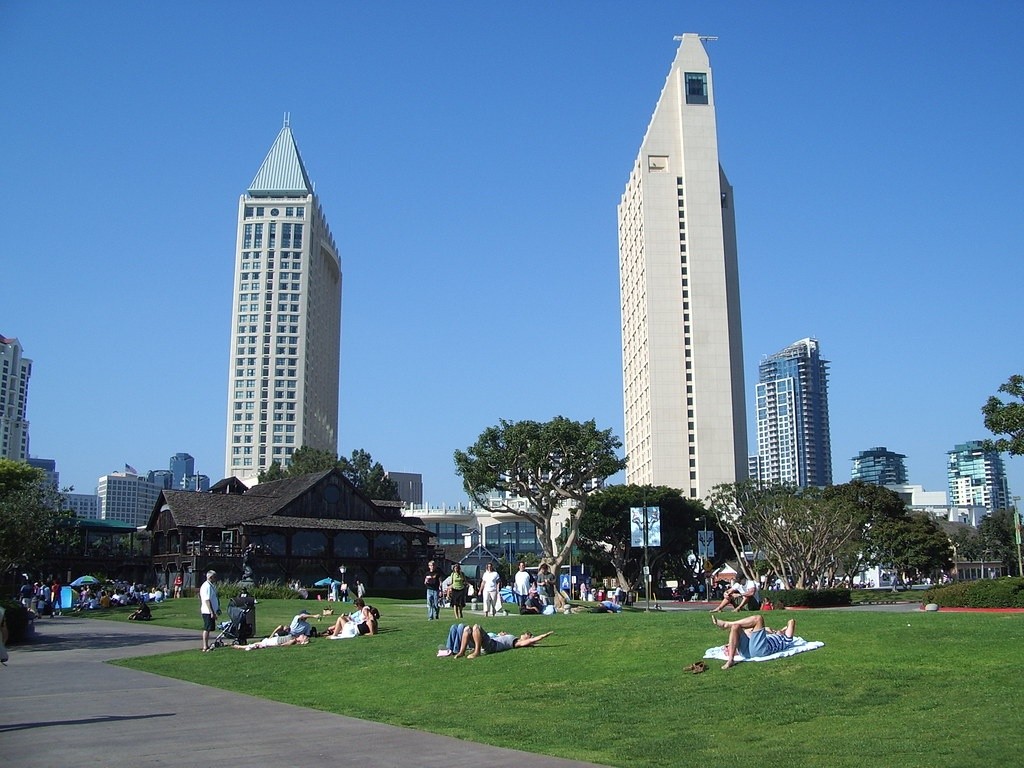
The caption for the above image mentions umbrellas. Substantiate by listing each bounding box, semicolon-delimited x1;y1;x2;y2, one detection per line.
69;574;100;590
315;577;342;597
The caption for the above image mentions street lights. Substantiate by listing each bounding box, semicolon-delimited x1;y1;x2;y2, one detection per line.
694;514;711;602
1012;495;1024;576
504;530;514;575
339;563;347;602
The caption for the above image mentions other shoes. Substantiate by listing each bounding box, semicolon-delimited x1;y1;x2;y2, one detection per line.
682;661;704;673
693;664;704;674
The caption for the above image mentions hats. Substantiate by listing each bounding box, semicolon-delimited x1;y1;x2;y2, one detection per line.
300;610;310;615
734;573;745;582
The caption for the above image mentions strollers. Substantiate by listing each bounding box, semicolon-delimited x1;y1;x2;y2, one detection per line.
208;595;257;649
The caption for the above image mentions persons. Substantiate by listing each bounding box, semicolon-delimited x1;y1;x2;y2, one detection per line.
200;570;219;652
424;560;996;669
0;571;183;666
230;579;380;651
